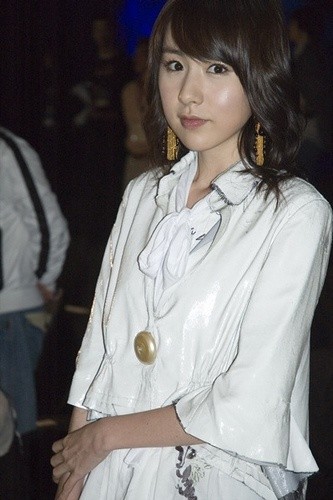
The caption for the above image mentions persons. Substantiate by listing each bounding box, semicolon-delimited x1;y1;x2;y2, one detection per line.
47;0;332;498
1;125;70;500
70;7;332;231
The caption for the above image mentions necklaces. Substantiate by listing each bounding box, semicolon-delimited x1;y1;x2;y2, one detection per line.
132;205;233;363
